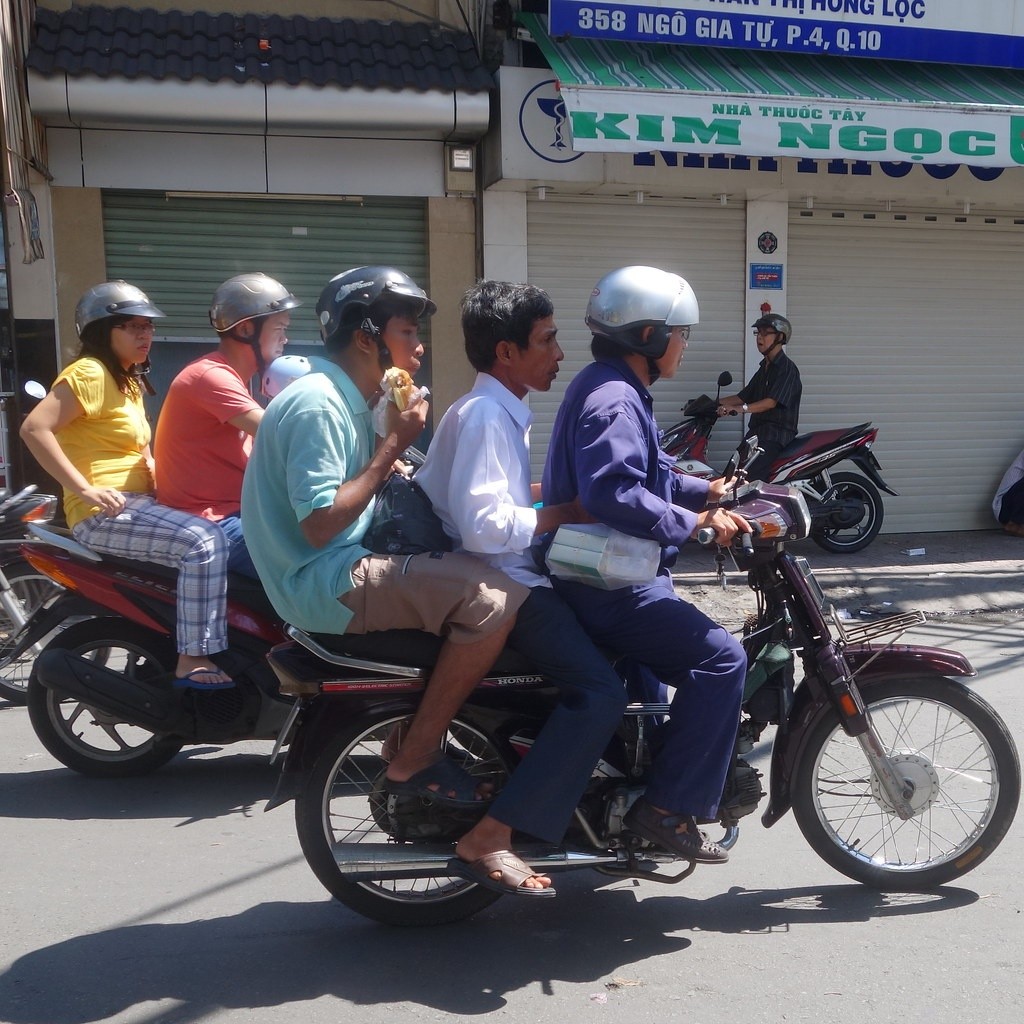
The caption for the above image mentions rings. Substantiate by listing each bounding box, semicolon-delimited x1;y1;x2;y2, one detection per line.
115;495;119;499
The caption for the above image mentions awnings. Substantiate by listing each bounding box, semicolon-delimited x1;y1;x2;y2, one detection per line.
519;14;1024;168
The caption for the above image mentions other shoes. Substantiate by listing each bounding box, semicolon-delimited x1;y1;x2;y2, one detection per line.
622;794;729;862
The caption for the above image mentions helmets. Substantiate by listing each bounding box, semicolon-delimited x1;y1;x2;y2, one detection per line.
260;354;311;400
751;314;791;345
209;272;301;332
584;265;699;336
74;280;167;341
317;264;437;344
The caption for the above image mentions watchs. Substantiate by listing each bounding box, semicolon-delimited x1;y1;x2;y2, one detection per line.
743;403;748;412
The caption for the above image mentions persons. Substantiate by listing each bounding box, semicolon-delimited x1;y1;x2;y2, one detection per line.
716;313;802;482
541;267;752;863
21;279;237;689
154;272;301;582
412;283;627;898
243;265;533;808
992;449;1024;537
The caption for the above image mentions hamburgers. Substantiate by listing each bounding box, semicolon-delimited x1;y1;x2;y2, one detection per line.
386;366;412;412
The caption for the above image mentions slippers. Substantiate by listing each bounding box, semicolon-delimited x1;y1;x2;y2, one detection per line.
384;764;498;808
447;849;557;898
170;663;236;691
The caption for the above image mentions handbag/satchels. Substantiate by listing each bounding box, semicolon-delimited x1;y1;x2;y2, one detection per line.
362;469;453;555
544;520;661;589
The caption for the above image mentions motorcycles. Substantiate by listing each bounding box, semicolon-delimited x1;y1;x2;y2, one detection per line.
262;398;1022;931
0;377;448;785
657;370;901;555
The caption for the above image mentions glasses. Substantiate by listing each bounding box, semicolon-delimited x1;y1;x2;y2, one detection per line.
671;326;691;341
753;330;777;337
111;321;155;335
